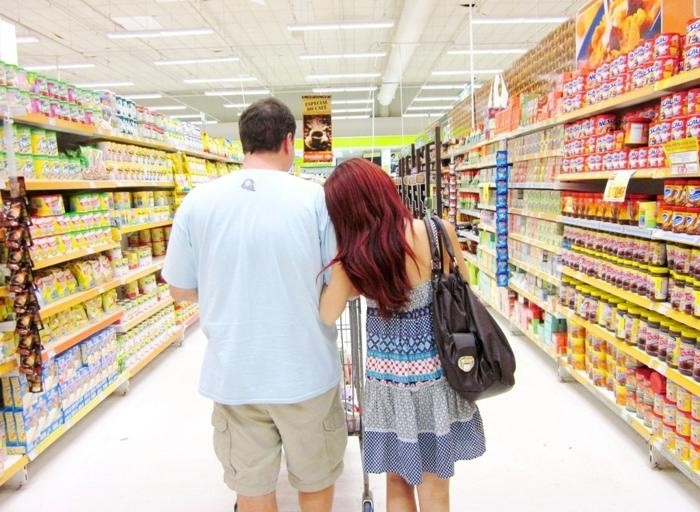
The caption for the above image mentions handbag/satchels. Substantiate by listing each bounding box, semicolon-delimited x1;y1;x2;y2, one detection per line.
423;209;520;404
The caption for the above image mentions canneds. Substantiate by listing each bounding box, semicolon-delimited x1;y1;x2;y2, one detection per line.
559;180;700;383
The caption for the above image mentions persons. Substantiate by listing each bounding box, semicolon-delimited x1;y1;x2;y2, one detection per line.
317;156;488;512
159;95;348;512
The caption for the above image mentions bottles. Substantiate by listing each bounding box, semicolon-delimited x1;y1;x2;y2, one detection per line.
454;123;483;212
559;224;700;384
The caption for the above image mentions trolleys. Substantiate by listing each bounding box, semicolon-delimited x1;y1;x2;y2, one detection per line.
233;288;376;512
